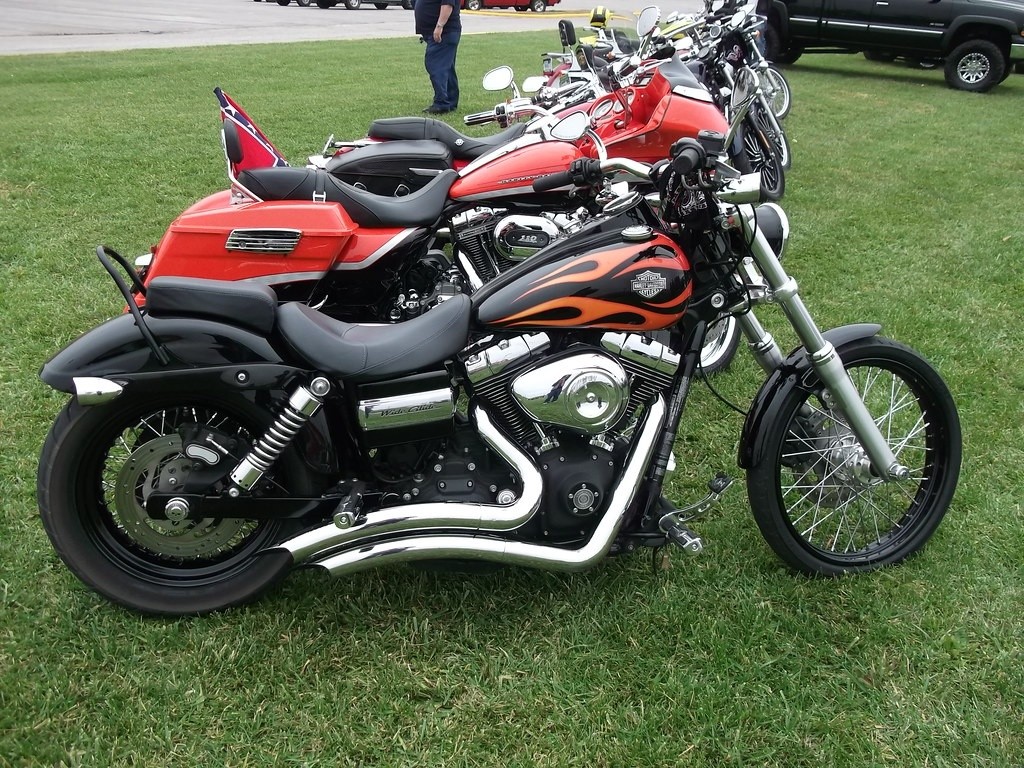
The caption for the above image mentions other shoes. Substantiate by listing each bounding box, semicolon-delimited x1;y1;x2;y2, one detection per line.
422;105;449;114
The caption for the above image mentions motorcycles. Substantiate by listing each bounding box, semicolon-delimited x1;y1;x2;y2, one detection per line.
32;66;968;619
123;0;795;378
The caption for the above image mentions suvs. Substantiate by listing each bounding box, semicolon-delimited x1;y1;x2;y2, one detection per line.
714;1;1023;92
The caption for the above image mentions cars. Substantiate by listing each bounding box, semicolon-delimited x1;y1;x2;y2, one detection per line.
456;0;560;13
309;0;413;11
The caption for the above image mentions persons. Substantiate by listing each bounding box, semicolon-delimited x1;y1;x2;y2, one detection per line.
415;0;462;114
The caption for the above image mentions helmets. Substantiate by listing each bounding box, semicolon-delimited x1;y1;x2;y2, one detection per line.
590;6;611;28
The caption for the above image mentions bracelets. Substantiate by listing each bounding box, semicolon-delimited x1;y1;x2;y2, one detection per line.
436;22;443;28
